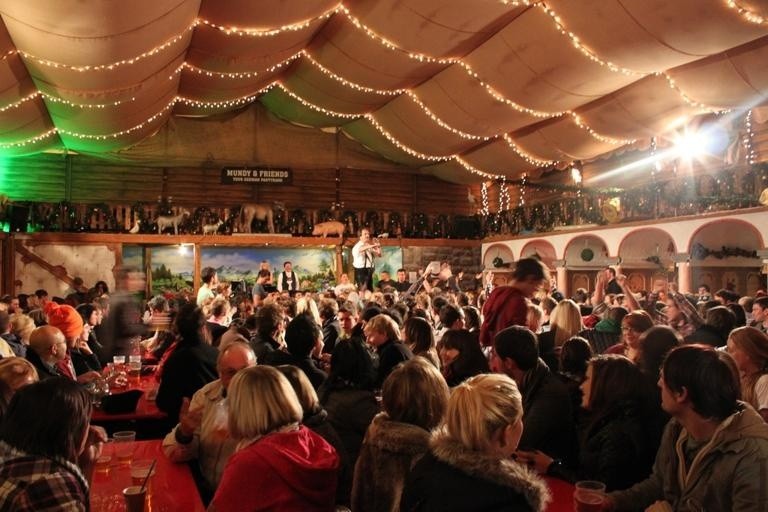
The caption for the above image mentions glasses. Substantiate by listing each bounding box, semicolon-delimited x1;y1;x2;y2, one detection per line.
620;326;642;334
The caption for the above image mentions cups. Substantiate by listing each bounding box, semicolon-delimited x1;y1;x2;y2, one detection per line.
574;481;606;511
107;354;142;382
213;403;229;439
96;431;158;512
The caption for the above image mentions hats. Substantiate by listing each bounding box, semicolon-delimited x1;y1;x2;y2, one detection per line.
43;301;84;339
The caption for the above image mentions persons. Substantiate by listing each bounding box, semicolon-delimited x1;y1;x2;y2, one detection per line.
352;227;382;291
2;259;767;512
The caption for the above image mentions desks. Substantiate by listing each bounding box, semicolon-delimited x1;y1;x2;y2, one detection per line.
89;363;170;439
539;474;614;512
88;438;207;512
